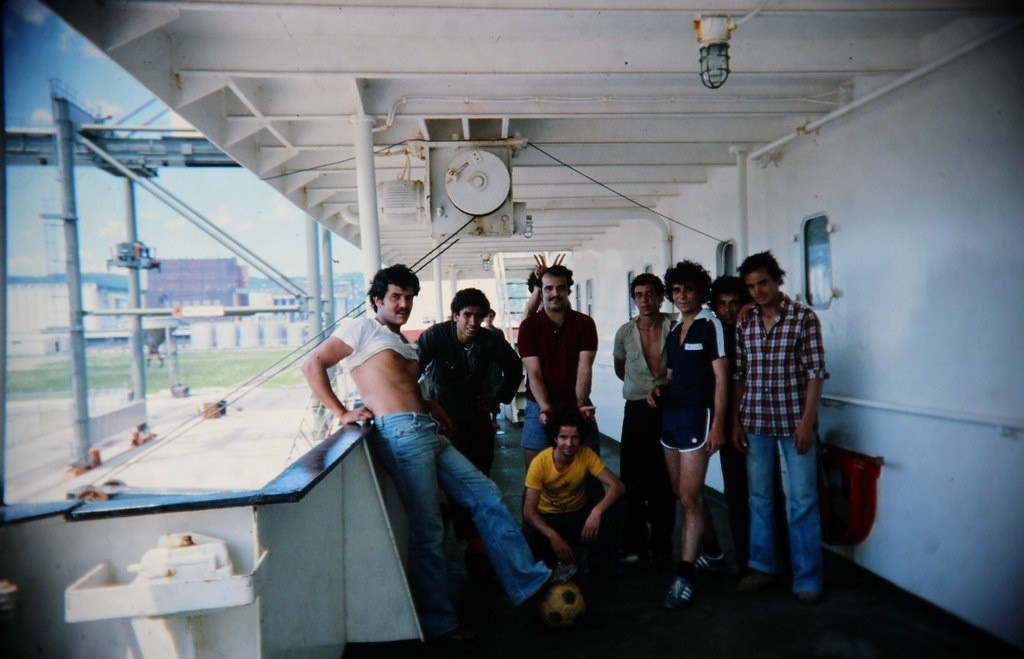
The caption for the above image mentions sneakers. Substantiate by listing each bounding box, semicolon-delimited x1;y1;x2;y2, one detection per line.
694;552;728;570
664;576;697;609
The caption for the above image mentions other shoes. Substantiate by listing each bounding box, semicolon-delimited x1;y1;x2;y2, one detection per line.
532;564;578;605
589;557;624;578
798;589;822;605
436;626;477;641
649;538;673;569
463;547;497;577
735;565;776;595
614;542;640;562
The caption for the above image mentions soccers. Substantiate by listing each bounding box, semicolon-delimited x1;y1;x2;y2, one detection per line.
537;582;586;628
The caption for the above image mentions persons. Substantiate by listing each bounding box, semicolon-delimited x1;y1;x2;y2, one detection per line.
482;310;504;428
523;414;625;567
523;253;572;318
646;258;730;611
411;288;523;565
301;263;578;646
613;274;677;557
733;247;830;604
518;266;601;474
705;272;792;571
